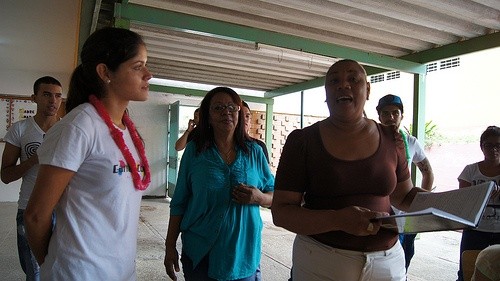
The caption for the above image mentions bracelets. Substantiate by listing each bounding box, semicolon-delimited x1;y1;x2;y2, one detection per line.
186;130;191;134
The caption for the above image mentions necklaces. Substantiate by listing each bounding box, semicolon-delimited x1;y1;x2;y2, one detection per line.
216;145;234;159
397;129;411;165
88;94;152;192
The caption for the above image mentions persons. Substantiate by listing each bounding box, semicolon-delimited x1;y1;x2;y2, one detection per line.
470;245;500;281
163;86;275;281
240;101;269;281
1;76;62;281
174;108;200;152
453;125;500;281
375;94;434;281
22;26;152;281
269;60;435;281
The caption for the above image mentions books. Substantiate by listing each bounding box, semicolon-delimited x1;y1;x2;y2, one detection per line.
371;181;494;236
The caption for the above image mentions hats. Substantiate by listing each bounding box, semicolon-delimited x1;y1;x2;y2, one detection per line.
376;94;404;113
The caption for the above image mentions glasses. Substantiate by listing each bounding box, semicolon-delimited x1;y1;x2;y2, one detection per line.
208;104;241;113
481;142;500;149
